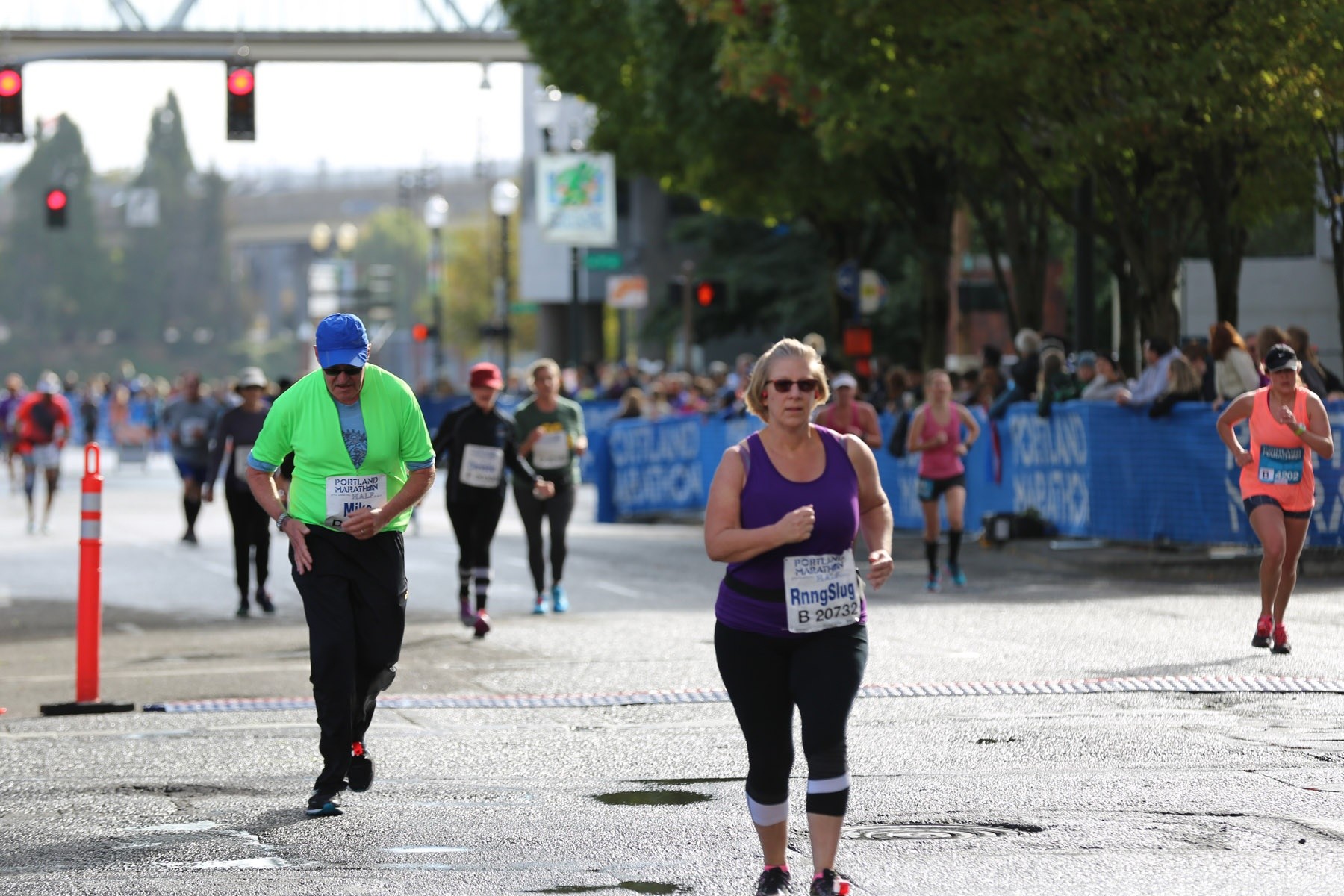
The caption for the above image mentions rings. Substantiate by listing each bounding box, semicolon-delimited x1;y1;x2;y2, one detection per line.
361;529;365;534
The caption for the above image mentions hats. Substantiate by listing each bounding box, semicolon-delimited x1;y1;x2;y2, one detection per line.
236;366;266;388
316;312;369;369
1264;344;1298;373
471;362;504;389
830;372;857;390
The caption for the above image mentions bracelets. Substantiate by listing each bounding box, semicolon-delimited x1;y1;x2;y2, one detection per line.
964;441;975;453
1295;423;1307;436
274;511;291;532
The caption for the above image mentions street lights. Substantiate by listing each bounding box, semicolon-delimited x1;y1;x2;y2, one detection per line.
423;194;448;374
489;178;522;393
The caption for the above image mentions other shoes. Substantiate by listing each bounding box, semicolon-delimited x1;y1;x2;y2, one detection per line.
551;583;569;613
460;600;474;626
345;734;375;792
946;557;968;587
182;528;196;542
926;568;943;591
237;600;249;616
306;776;344;817
472;612;491;638
256;589;274;612
533;592;548;615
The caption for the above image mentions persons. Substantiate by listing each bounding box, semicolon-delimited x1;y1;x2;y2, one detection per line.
203;368;278;618
509;359;588;615
1216;343;1335;654
906;370;981;593
704;340;894;896
430;363;555;639
246;316;436;817
0;320;1344;639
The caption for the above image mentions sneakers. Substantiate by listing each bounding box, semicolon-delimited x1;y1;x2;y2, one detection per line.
1250;614;1274;647
809;868;854;896
754;866;797;896
1269;627;1293;654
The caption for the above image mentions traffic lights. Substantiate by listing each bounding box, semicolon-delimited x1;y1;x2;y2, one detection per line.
692;274;727;309
227;63;256;141
0;61;24;135
410;320;432;344
45;186;66;227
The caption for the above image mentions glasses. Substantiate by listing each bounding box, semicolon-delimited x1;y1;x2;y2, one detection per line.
763;377;819;393
322;364;363;376
